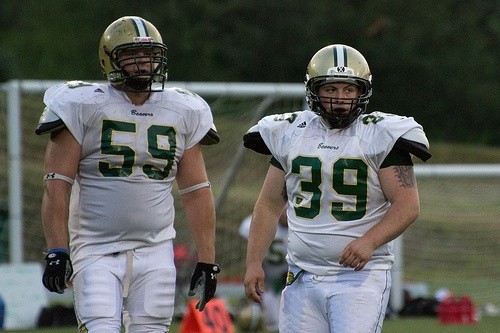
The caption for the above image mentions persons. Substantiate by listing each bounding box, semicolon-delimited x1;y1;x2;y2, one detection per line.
35;16;220;333
239;45;432;333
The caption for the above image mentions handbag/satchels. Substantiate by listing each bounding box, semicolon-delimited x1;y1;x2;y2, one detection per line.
439;297;476;325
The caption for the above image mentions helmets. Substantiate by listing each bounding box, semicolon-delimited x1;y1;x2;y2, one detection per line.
304;44;372;101
98;16;165;84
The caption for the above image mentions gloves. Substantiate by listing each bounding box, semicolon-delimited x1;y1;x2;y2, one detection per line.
188;262;221;311
42;248;73;294
